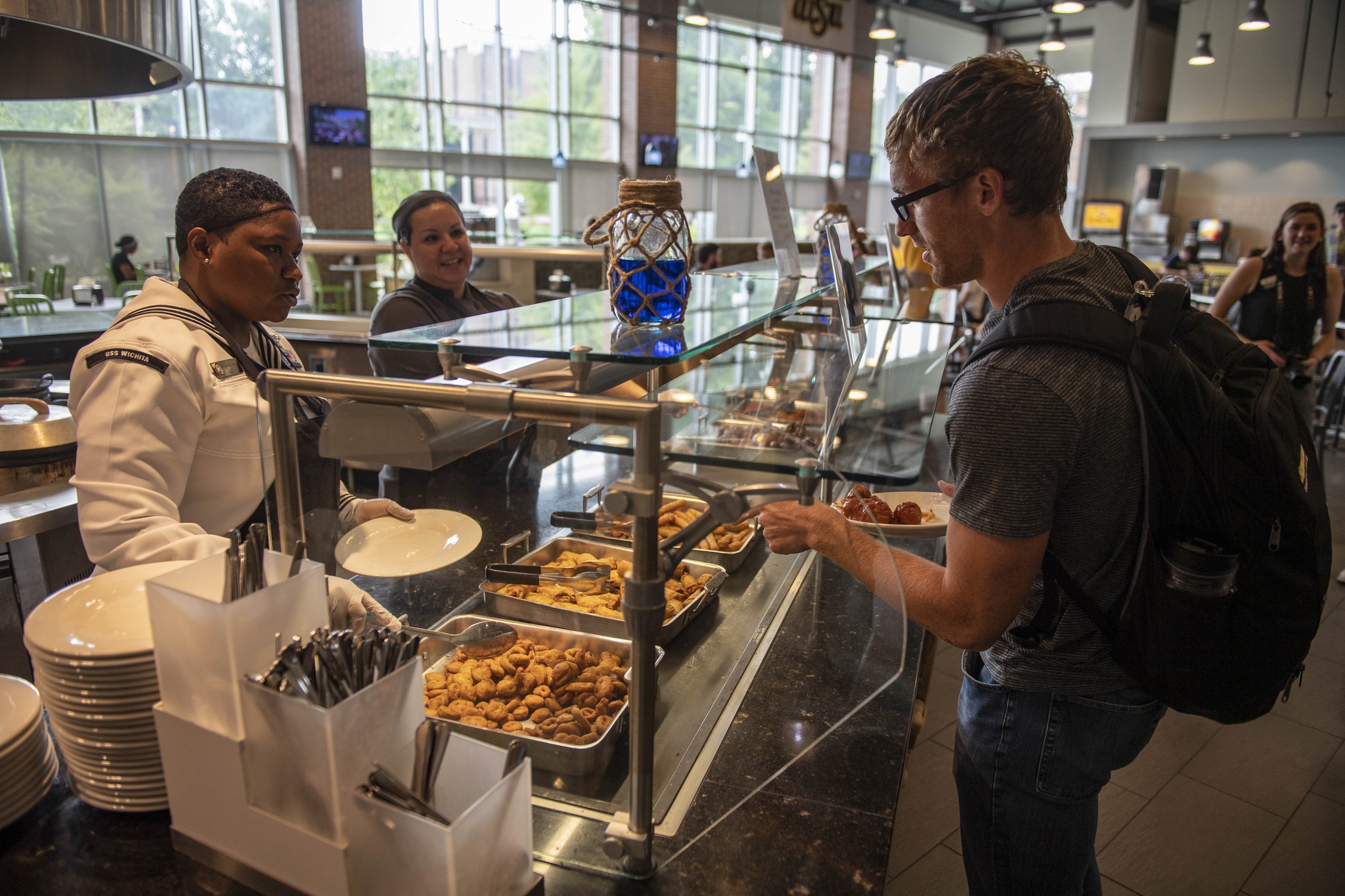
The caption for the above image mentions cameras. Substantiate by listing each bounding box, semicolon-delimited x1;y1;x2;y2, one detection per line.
1279;351;1311;390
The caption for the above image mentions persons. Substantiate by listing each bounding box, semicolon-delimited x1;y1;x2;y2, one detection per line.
1203;204;1344;446
366;190;719;523
109;236;138;283
61;166;421;653
1157;245;1203;277
748;53;1175;896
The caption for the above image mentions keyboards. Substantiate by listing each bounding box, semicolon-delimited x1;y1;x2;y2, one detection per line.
1086;236;1123;248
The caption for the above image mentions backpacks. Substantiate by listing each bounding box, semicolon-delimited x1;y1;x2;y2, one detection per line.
962;246;1332;725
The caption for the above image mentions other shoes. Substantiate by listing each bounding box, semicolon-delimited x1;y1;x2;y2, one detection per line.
1336;570;1345;584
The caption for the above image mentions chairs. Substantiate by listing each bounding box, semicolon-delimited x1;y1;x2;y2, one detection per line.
4;264;66;316
365;258;401;310
307;256;350;314
106;263;144;307
1314;351;1345;472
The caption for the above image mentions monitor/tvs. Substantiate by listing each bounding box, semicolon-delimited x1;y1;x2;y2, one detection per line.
637;132;677;168
1080;199;1123;233
306;104;370;146
845;152;872;180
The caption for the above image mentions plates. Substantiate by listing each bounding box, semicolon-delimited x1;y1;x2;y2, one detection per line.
0;560;237;829
332;509;484;577
829;491;954;539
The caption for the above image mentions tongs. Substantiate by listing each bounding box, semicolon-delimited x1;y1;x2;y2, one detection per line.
550;510;634;539
485;561;612;595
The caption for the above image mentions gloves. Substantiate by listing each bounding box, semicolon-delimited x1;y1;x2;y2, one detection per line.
349;498;414;528
323;574;402;634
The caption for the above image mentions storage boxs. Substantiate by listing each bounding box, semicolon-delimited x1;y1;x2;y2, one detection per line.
145;543;534;896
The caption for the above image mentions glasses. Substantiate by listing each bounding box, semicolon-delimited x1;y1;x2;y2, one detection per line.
890;167;982;221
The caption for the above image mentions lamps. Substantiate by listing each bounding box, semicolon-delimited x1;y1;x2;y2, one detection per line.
1237;0;1270;31
1038;0;1085;52
869;6;896;40
684;0;708;26
1188;0;1215;65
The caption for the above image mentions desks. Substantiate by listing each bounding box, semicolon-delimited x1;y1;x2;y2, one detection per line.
329;263;376;317
5;297;134;316
